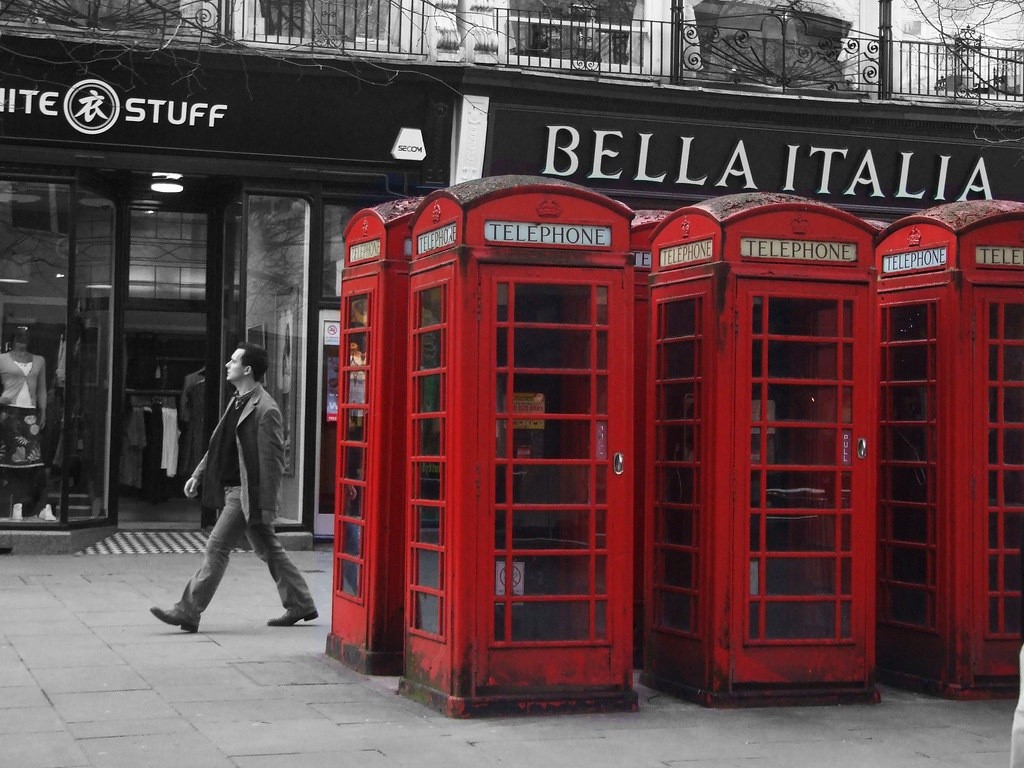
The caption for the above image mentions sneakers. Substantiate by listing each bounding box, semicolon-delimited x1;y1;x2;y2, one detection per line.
12;503;23;520
39;504;56;520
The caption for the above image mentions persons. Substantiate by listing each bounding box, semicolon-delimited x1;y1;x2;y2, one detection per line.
0;322;56;521
344;342;363;428
148;344;318;632
180;365;206;472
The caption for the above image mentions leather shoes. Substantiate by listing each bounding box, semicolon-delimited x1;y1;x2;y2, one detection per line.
150;607;199;632
268;611;318;626
